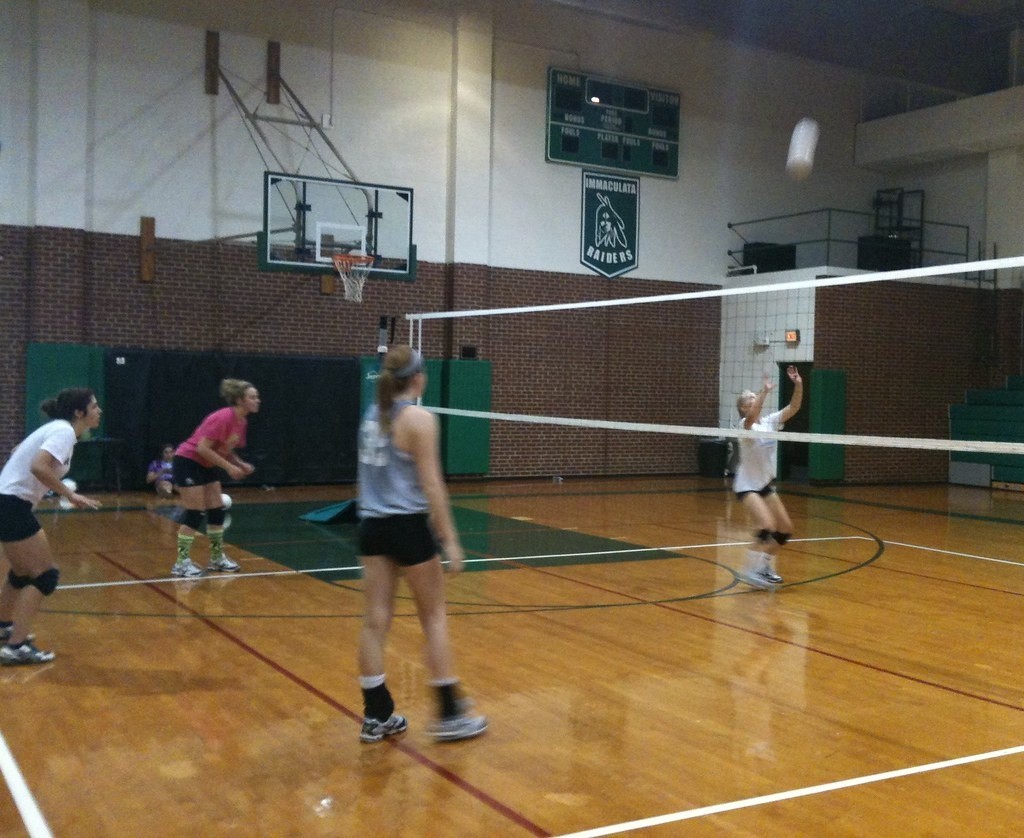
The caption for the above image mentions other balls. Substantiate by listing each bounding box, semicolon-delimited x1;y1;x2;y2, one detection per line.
220;493;232;510
62;478;77;492
786;154;813;181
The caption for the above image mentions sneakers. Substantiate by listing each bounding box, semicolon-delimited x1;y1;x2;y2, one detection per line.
754;567;782;583
0;642;56;665
171;558;203;578
431;715;488;742
0;624;38;643
359;715;409;744
209;553;241;572
736;571;773;591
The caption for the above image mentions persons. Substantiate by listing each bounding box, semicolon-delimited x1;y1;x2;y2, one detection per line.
733;366;803;592
172;377;261;576
0;389;103;663
355;345;489;743
146;444;182;499
